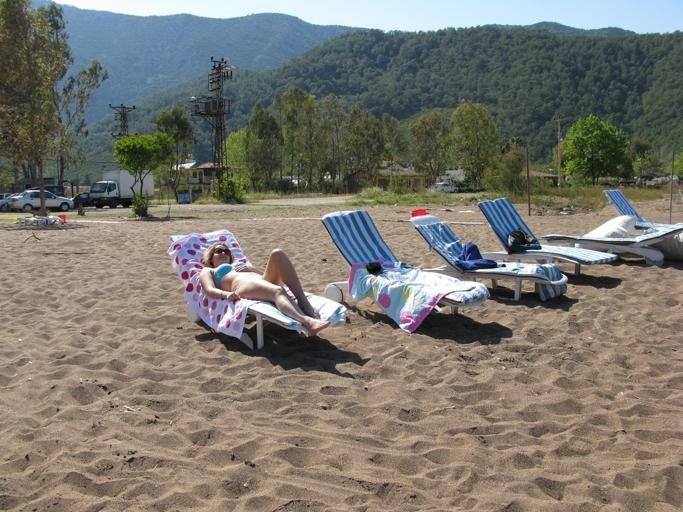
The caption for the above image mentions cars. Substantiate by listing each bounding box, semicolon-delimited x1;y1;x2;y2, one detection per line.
72;192;94;207
428;183;458;193
0;188;74;212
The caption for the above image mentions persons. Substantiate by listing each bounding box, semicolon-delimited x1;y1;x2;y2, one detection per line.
200;242;330;334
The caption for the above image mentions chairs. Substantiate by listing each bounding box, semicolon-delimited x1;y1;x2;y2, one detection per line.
320;209;490;315
477;197;619;277
540;222;683;265
410;211;568;301
603;189;683;232
169;229;347;351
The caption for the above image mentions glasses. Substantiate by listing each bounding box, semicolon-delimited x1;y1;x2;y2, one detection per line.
214;247;232;255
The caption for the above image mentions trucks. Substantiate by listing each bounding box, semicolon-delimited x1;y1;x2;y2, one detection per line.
89;168;155;208
